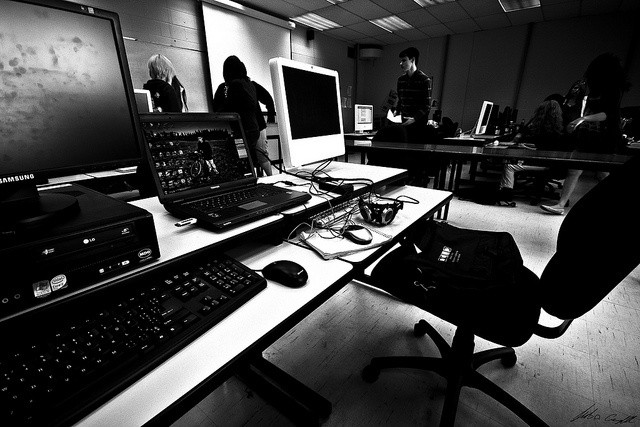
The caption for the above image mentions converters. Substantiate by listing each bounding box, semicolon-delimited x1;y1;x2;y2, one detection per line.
318;179;355;195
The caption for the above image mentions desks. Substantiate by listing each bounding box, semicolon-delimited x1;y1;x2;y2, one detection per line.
1;161;452;426
444;128;528;194
344;131;378;164
345;142;639;220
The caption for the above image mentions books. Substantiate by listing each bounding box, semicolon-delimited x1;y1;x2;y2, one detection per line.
300;223;392;260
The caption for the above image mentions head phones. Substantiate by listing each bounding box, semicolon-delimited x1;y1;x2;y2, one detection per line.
358;199;398;225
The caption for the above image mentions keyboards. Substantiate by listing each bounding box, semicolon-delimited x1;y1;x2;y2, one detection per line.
0;247;266;427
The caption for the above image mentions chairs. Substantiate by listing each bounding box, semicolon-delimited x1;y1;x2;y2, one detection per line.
515;165;568;206
361;152;639;427
441;116;453;137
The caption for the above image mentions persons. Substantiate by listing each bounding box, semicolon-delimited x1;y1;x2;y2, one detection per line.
212;62;276;176
142;54;189;112
544;93;565;108
194;136;219;174
393;47;432;188
540;52;621;215
496;99;567;208
220;55;260;175
561;80;588;123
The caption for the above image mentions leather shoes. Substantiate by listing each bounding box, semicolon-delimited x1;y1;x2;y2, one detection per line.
542;204;565;215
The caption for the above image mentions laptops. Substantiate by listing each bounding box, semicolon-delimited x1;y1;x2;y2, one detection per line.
137;112;311;232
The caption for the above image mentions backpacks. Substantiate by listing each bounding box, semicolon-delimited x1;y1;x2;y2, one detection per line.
403;220;524;315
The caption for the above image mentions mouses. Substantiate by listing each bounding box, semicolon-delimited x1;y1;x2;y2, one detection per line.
342;225;373;245
262;260;309;288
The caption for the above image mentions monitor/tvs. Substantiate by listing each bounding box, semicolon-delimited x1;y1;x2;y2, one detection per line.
354;104;374;135
269;56;346;172
133;87;154;116
0;1;149;226
456;101;494;140
